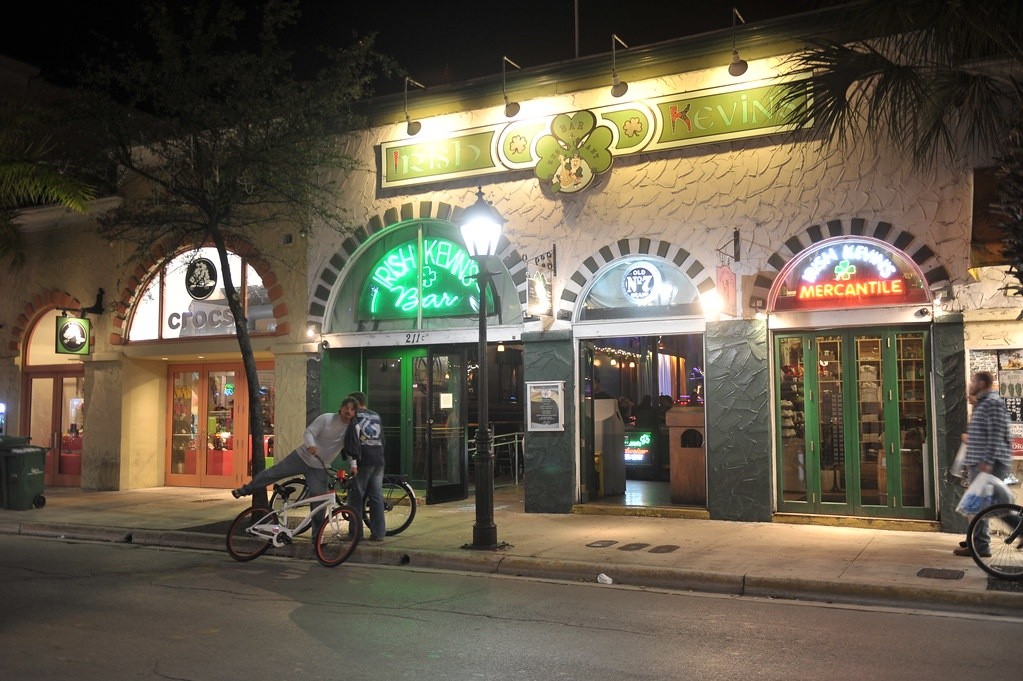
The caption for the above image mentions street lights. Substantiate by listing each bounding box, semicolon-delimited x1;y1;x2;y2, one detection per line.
458;185;512;552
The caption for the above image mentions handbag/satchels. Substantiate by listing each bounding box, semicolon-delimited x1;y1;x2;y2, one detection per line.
948;442;968;480
954;472;1017;520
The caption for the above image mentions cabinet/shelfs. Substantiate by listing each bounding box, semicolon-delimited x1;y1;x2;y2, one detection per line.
784;337;925;488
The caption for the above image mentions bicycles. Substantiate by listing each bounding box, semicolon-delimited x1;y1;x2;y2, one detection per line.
226;454;418;568
969;503;1023;580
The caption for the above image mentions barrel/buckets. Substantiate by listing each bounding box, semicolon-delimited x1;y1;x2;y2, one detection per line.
878;449;919;506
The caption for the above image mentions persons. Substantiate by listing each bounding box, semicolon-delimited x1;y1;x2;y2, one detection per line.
619;392;700;428
231;397;361;561
593;366;613;399
953;370;1023;557
340;391;388;542
444;411;460;482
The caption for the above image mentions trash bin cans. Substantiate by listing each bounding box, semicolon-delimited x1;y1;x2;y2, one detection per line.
665;407;705;507
877;449;922;507
0;435;46;511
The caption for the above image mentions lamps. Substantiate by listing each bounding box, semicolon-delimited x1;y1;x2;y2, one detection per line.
501;56;522;117
611;34;629;97
403;76;425;135
658;336;665;349
728;8;749;76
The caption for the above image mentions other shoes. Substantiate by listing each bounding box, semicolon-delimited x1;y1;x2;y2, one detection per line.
340;535;366;544
368;535;387;542
231;488;243;499
954;546;992;558
1016;536;1023;549
959;542;969;548
